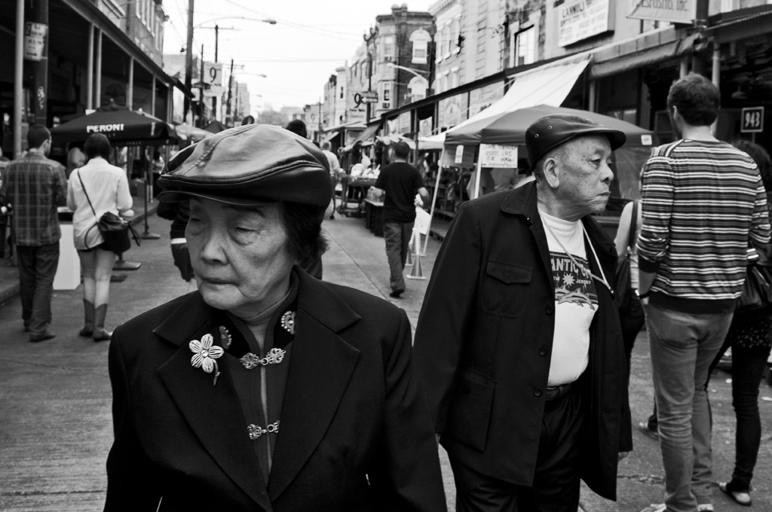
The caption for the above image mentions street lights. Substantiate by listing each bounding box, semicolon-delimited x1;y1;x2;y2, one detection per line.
227;72;267;121
385;61;430;89
187;0;278;124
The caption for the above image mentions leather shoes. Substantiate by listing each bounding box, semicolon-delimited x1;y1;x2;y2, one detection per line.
28;331;55;342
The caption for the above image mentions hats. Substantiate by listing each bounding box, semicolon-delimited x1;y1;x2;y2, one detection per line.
157;123;334;213
525;115;627;169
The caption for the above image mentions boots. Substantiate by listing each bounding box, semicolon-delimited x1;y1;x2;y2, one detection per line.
79;299;112;342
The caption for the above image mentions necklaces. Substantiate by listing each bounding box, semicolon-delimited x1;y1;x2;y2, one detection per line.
538;206;616;295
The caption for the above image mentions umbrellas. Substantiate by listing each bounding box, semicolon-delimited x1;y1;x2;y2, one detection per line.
50;97;217;263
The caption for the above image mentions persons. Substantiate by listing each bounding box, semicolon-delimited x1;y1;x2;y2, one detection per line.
612;198;659;442
320;140;347;195
413;113;634;511
67;133;134;342
373;142;427;299
635;68;772;512
101;121;450;512
0;123;68;344
704;138;772;506
67;146;87;177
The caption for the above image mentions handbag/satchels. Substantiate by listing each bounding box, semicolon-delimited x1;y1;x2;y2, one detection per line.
96;212;131;253
